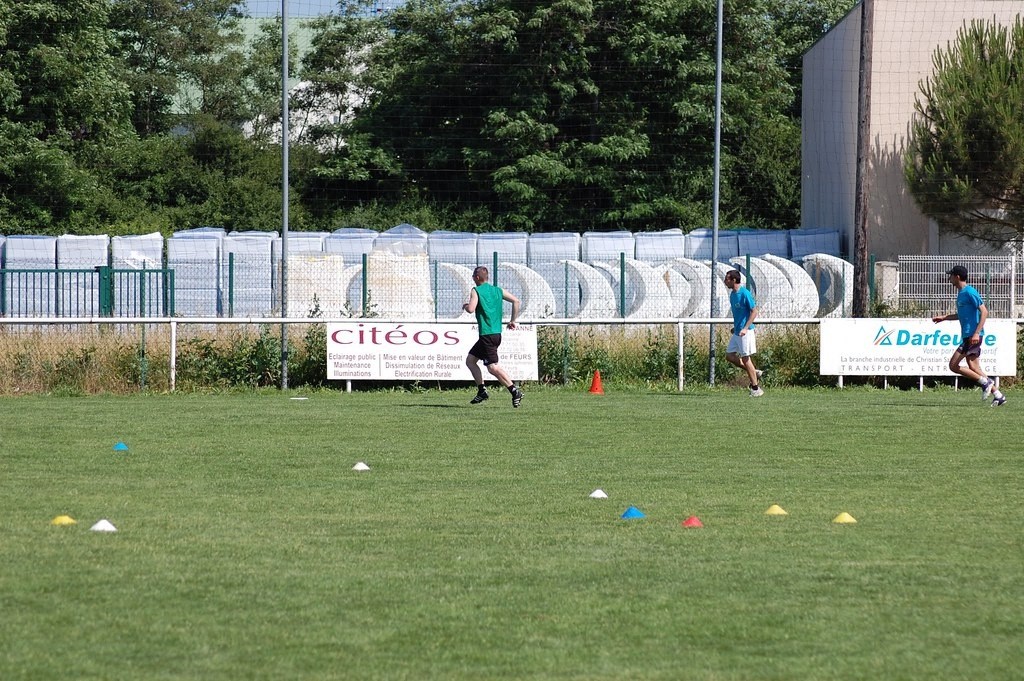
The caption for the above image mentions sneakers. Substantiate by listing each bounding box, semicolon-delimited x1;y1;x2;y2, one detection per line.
471;386;488;403
748;387;764;398
511;387;525;409
755;369;764;379
981;378;995;401
991;395;1007;408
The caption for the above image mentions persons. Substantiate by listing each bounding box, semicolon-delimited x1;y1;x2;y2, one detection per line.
461;266;524;409
932;265;1007;408
723;270;764;398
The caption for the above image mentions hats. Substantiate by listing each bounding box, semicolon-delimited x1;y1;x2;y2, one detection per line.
946;265;968;277
